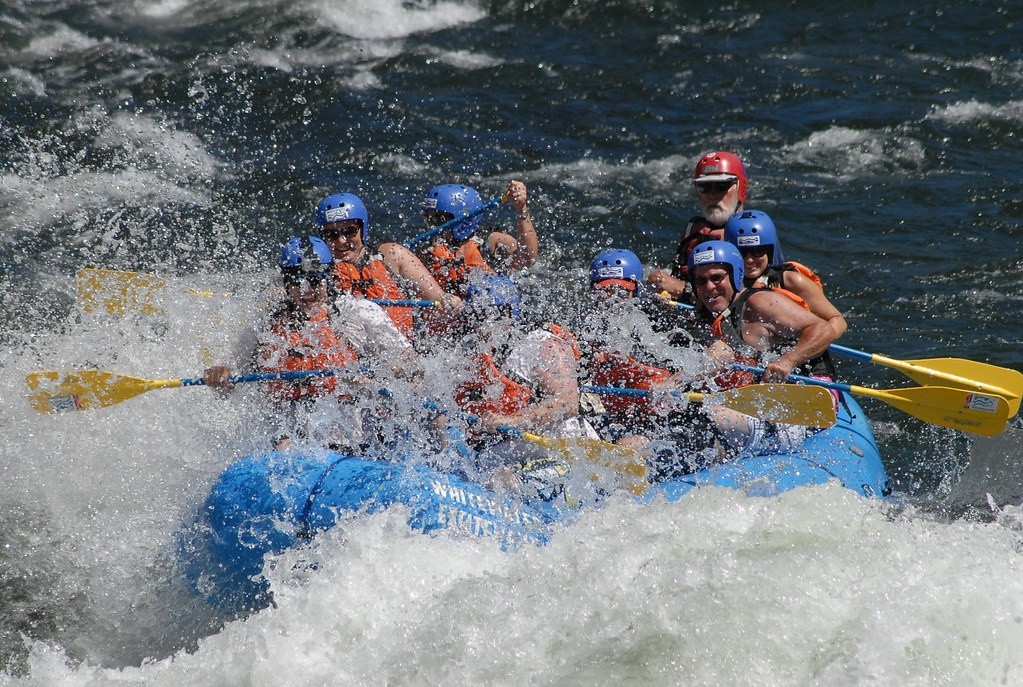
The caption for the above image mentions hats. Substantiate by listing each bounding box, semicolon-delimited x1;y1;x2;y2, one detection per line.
691;173;738;183
595;278;635;291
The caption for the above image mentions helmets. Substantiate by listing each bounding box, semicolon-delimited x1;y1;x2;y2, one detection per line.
694;151;748;205
461;274;522;322
277;235;333;268
418;183;484;241
687;240;745;299
588;248;644;306
724;209;780;267
317;191;369;245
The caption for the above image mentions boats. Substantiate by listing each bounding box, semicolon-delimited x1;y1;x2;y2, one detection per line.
172;359;895;630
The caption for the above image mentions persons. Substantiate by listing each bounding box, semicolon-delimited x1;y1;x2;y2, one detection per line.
206;152;846;492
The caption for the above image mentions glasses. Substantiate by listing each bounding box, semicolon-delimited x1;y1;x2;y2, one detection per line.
737;245;770;260
696;181;738;194
421;212;452;227
280;265;333;288
319;225;361;242
693;272;730;288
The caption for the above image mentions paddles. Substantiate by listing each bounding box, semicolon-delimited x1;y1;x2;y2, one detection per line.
74;268;441;317
659;290;1023;419
379;387;648;496
26;365;371;414
731;361;1010;437
581;385;837;429
402;194;510;249
